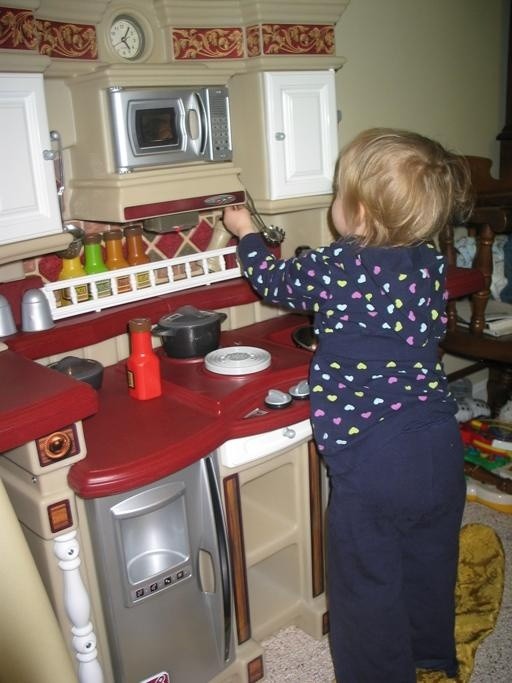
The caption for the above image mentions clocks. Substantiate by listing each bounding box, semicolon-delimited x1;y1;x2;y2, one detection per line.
106;12;154;63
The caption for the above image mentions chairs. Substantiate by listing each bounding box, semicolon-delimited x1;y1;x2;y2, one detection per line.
427;156;512;373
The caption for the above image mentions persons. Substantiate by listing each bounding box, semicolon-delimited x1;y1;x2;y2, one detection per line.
222;128;477;682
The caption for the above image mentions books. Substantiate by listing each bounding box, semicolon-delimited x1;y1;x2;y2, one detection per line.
452;299;512;337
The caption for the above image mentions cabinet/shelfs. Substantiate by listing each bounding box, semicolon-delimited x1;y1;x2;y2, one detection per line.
217;420;328;682
0;53;75;263
232;53;342;213
